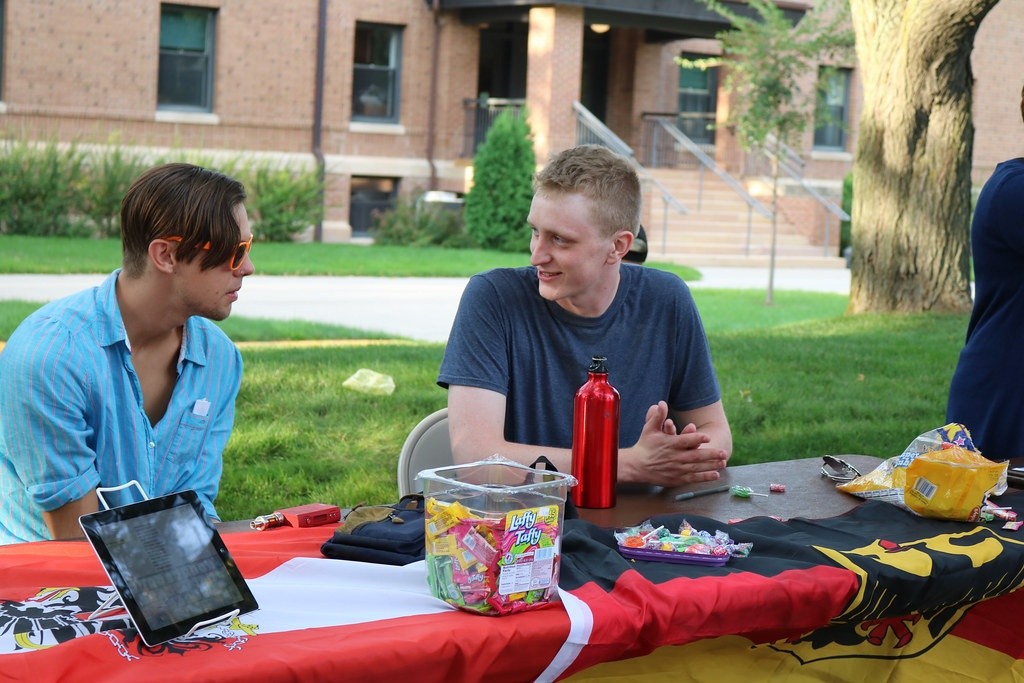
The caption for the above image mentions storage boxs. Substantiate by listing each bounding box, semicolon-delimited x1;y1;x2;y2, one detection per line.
413;455;578;616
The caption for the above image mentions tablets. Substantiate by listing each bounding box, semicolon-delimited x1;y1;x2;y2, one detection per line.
78;490;259;647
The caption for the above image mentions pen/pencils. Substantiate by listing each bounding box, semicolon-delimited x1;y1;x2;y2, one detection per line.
675;484;731;502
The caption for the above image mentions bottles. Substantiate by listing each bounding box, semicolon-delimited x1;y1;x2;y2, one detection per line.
571;356;621;509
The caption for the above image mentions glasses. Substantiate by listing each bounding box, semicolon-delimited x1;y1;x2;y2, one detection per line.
821;455;861;483
159;233;253;270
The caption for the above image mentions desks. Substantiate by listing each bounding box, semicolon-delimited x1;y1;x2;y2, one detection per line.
1;454;1024;683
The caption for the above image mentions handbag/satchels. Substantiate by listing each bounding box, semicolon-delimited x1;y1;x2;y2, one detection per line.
319;492;425;565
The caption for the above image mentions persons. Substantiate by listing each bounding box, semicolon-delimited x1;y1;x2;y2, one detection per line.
437;144;732;492
946;85;1024;462
0;163;255;545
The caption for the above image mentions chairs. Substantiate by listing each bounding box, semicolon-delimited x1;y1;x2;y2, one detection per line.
396;408;453;499
